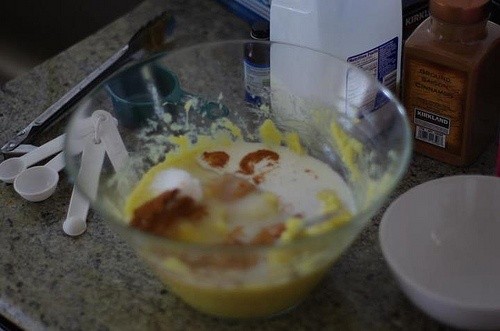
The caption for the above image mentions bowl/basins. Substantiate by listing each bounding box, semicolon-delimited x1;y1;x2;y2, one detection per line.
105;63;229;128
378;175;500;330
63;38;412;322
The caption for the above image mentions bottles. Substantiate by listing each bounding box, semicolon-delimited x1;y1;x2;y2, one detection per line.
401;1;499;169
243;20;271;106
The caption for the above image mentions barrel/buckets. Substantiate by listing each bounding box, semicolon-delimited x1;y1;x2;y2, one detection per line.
270;0;404;142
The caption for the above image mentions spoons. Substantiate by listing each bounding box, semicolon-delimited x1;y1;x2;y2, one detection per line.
0;118;95;184
13;117;118;201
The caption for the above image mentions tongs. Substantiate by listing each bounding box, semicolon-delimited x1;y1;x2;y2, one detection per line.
2;8;175;168
